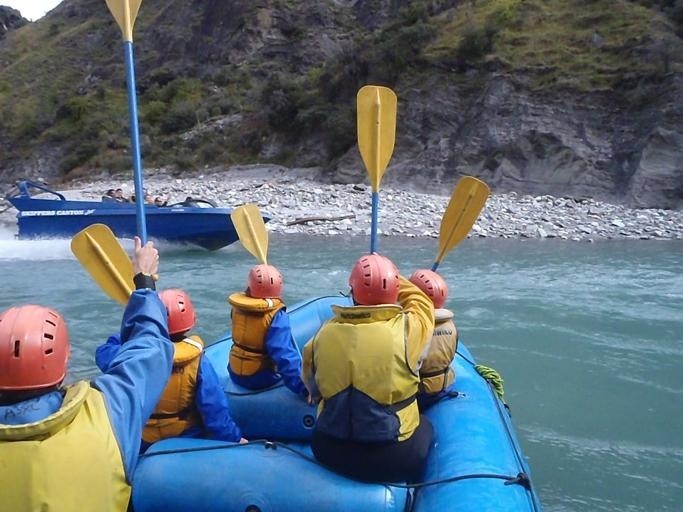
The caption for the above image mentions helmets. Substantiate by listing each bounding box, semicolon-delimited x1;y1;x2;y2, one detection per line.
247;263;283;300
158;288;196;335
408;268;449;309
348;253;401;306
0;303;70;394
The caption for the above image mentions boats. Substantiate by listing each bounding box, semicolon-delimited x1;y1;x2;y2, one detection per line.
7;179;271;250
128;295;541;511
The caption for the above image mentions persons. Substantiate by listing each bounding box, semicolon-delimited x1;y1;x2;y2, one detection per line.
225;263;308;402
300;252;434;482
93;285;250;454
104;186;169;209
0;235;175;510
407;267;460;413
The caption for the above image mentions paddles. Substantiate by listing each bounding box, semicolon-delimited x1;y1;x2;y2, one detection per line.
433;176;489;272
231;203;304;362
357;86;398;253
71;223;136;305
106;0;160;281
